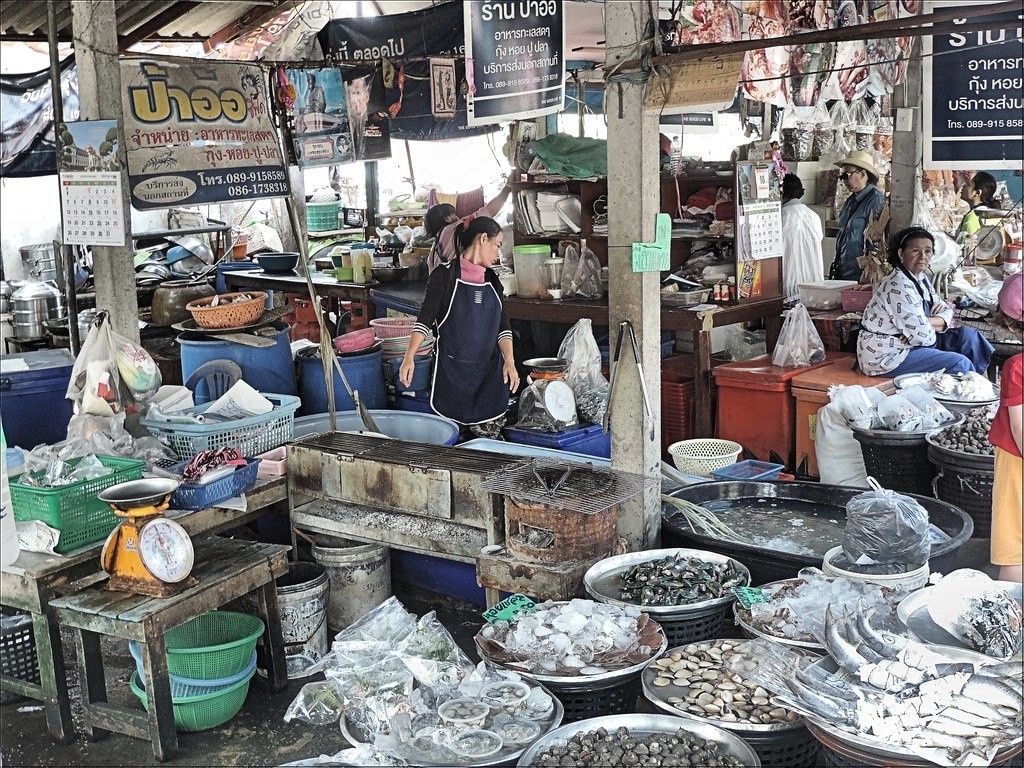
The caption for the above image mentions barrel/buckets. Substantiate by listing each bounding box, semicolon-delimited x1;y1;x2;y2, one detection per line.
850;379;996;539
294;345;388;416
239;561;330;679
311;533;393;632
512;244;551;299
176;320;300;419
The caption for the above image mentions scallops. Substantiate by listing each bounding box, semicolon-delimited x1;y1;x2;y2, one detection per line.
649;637;822;724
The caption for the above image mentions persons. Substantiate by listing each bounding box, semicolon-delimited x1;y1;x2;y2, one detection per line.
988;271;1024;583
954;171;1001;241
298;74;326;116
856;227;995;381
295;141;301;159
400;170;521;439
781;173;824;303
828;150;888;281
337;136;349;154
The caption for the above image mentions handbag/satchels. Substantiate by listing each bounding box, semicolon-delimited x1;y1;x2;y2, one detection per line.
771;302;826;367
64;311;163;412
842;476;931;565
829;258;841;280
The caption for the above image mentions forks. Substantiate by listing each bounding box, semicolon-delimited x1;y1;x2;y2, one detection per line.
602;319;656;442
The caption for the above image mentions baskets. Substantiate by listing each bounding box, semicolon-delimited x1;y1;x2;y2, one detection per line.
668;438;740;480
138;391;302;464
162;451;261;509
838;284;872;312
186;291;268;328
128;608;265;733
332;323;375;353
369;318;435;354
708;452;784;483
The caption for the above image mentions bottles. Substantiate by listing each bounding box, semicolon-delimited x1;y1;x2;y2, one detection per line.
352;248;372;283
965;234;978;269
669;135;682;178
721;284;730;301
727;276;737;301
714;284;721;300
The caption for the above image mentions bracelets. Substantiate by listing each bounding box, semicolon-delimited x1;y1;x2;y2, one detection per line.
504;184;512;188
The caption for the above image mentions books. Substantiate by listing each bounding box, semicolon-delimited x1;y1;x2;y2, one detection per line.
735;159;784;261
54;119;128;246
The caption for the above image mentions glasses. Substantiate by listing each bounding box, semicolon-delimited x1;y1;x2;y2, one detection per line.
838;167;863;183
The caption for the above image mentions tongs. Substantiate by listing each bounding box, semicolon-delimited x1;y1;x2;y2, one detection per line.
533;463;572;498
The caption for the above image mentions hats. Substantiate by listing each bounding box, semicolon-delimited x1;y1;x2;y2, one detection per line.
833;150;879;186
997;273;1022;321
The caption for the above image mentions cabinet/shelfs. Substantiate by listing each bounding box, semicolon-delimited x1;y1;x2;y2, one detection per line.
501;176;785;440
782;161;837;277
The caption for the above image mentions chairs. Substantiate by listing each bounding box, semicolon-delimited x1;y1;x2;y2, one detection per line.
185;360;242;405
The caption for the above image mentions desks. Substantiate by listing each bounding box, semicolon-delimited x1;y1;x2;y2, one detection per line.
47;534;294;763
0;469;288;747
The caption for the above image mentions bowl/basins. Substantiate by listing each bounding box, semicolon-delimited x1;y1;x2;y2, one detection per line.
254;252;300;276
272;547;1024;768
370;267;409;284
661;477;974;588
134;235;215;286
293;408;460;446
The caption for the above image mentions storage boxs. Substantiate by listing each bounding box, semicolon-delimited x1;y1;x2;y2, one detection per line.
676;322;744;354
660;354;734;457
797;280;858;310
7;454;146;553
504;416;611;457
712;349;898;479
138;392;302;463
246;223;283;256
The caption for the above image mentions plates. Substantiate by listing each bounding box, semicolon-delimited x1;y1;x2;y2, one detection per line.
850;408;966;440
893;372;1000;406
715;171;734;176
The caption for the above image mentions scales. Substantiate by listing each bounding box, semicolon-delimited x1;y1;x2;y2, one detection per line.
972;209;1014;266
98;478;200;599
515;356;580;433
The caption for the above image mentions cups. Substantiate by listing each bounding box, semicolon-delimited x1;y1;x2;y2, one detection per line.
544;256;564;299
